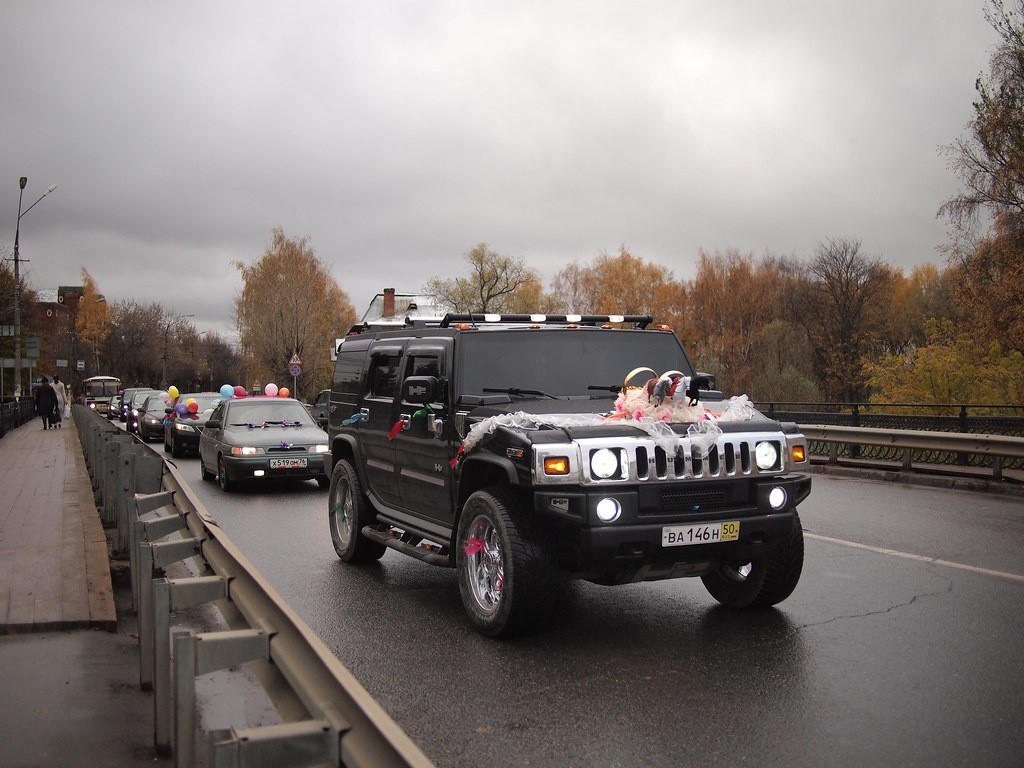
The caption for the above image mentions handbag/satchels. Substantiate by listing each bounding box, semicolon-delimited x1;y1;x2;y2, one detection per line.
63;403;70;418
50;406;62;424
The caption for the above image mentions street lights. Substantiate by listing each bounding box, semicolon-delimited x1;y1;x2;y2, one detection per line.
14;176;28;398
160;314;195;388
191;331;205;390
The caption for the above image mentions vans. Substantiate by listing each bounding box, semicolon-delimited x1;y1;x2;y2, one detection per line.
81;376;123;414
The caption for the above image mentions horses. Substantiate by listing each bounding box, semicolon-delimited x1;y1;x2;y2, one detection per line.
647;376;711;407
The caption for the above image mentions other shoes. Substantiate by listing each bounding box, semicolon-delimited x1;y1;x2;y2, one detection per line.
49;425;52;429
44;425;47;429
54;425;57;428
59;424;61;428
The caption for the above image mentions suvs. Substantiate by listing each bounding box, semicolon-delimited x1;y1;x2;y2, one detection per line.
329;311;811;643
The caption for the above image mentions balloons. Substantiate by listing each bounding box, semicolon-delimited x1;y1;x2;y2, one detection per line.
168;386;179;398
159;392;169;401
265;383;278;397
279;388;290;397
186;398;196;407
119;390;123;395
175;403;189;415
220;385;234;398
234;387;246;397
188;402;199;413
166;397;175;407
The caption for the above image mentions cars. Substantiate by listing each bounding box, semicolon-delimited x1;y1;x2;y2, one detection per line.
198;396;330;492
164;392;223;458
136;395;167;441
107;395;121;420
116;388;153;423
126;390;167;432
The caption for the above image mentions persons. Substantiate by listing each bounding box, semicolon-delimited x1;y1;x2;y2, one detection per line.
33;376;59;430
49;375;68;429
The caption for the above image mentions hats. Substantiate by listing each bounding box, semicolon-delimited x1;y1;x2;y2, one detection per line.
41;377;49;383
